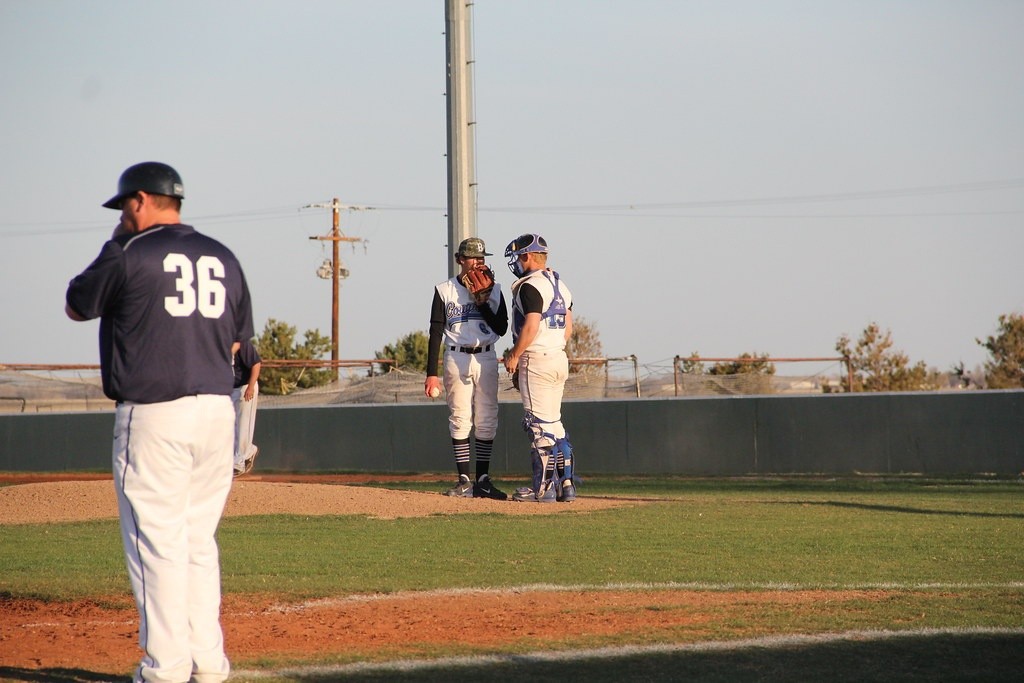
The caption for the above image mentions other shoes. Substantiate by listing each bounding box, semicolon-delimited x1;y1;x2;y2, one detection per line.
244;446;260;474
232;468;245;478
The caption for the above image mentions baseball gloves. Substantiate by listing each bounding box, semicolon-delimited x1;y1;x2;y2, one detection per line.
512;369;519;390
461;265;494;302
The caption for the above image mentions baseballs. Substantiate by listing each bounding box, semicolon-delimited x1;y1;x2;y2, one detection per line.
431;387;440;398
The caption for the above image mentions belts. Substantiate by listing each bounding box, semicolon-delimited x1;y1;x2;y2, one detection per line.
450;345;491;354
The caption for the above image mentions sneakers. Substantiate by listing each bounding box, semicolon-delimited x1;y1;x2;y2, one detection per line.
473;474;508;500
511;487;558;502
557;482;577;502
446;474;474;497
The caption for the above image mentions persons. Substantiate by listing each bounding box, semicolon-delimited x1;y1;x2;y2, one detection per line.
424;238;509;500
232;344;262;477
503;234;576;502
66;160;253;682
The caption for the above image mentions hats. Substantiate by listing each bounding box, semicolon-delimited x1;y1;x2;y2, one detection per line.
512;234;548;255
455;237;494;258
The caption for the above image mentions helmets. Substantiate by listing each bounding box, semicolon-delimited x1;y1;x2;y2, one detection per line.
101;162;186;210
